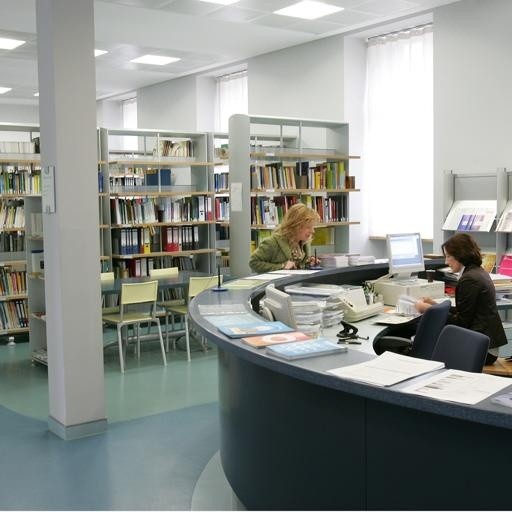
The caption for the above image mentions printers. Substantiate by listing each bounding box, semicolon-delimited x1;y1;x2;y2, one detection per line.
333;282;383;323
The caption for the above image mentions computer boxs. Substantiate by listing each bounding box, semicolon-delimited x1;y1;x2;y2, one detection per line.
365;279;446;308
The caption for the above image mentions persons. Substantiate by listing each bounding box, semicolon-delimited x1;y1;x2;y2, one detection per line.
418;232;508;366
247;202;322;274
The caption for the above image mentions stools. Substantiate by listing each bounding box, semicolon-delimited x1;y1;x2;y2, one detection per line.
482;358;512;377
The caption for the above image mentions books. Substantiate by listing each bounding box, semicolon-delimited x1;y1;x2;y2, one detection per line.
1;160;42;252
217;256;229;268
216;223;229;240
163;256;197;273
478;252;496;273
111;195;199;224
249;143;355;257
265;338;348;361
158;287;186;301
101;294;119;308
214;173;229;194
217;321;295;338
497;255;512;277
111;178;135;193
241;331;312;350
216;199;229;220
1;263;29;330
155;139;194;158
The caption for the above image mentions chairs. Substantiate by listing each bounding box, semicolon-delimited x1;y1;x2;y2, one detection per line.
149;267;186;353
431;324;491;372
102;280;168;373
164;274;223;365
99;271;130;353
375;300;451;360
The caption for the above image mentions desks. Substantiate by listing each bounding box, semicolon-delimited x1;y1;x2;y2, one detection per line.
104;268;207;349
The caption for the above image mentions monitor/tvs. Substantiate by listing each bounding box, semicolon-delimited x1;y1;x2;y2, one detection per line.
261;283;296;329
385;233;427;280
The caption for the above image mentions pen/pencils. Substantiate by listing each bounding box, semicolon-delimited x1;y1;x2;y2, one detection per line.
314;248;316;265
217;263;221;285
338;341;362;345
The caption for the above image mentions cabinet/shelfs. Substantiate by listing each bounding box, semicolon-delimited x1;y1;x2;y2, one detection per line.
100;126;230;322
441;167;512;275
230;116;361;261
0;120;50;366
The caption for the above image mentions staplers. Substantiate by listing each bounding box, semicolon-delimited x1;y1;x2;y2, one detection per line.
336;320;359;337
